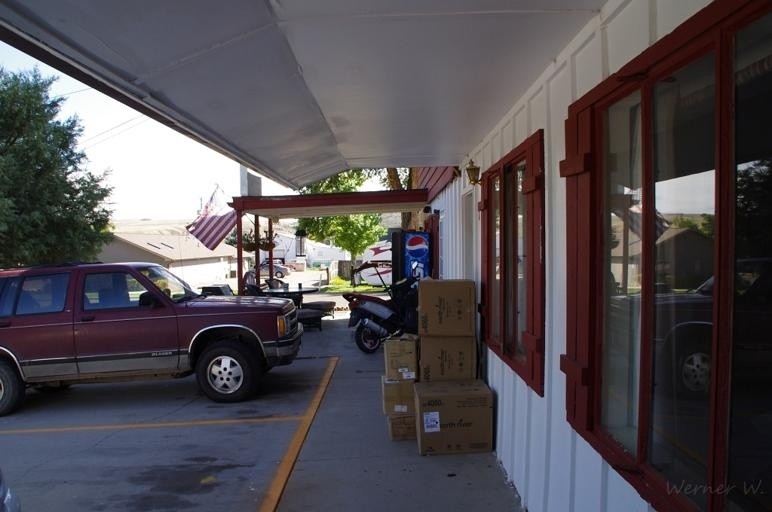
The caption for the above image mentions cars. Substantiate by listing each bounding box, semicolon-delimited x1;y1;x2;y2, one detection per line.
248;264;291;279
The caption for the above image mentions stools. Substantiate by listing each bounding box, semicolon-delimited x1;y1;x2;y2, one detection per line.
296;300;336;331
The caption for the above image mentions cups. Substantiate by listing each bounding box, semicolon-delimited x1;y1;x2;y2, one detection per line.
283;282;290;290
298;282;303;288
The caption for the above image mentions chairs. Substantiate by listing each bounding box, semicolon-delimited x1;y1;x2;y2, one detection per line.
83;288;118;310
243;278;289;296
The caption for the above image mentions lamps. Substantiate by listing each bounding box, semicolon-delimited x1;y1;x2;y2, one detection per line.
464;157;481;185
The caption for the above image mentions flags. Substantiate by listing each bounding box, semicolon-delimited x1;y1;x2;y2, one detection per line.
185;187;246;251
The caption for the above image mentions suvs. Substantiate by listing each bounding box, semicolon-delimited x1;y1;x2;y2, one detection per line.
598;255;770;397
1;262;308;416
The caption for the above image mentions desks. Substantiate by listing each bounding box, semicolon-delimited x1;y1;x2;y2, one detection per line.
257;287;319;309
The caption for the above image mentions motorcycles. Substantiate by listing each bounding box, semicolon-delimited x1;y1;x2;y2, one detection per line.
343;259;423;353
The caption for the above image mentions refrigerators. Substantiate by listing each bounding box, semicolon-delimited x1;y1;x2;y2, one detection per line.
391;229;433;291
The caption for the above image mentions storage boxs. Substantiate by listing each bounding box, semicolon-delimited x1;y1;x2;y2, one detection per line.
380;278;495;458
